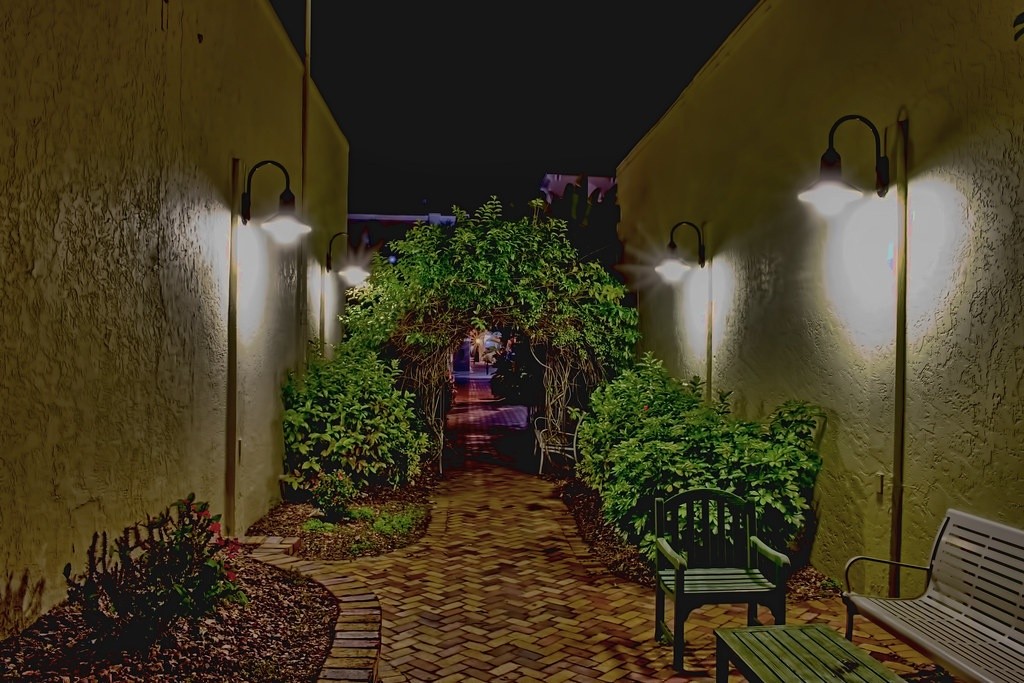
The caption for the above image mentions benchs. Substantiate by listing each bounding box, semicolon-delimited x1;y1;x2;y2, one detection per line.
839;506;1024;683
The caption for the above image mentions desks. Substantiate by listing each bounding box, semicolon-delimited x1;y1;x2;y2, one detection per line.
711;622;910;683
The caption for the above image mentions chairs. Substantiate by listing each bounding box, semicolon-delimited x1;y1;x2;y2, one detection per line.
532;405;587;475
651;487;792;673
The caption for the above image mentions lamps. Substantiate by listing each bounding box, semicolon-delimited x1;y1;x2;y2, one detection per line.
653;219;706;272
326;231;372;278
793;112;894;206
240;157;314;249
